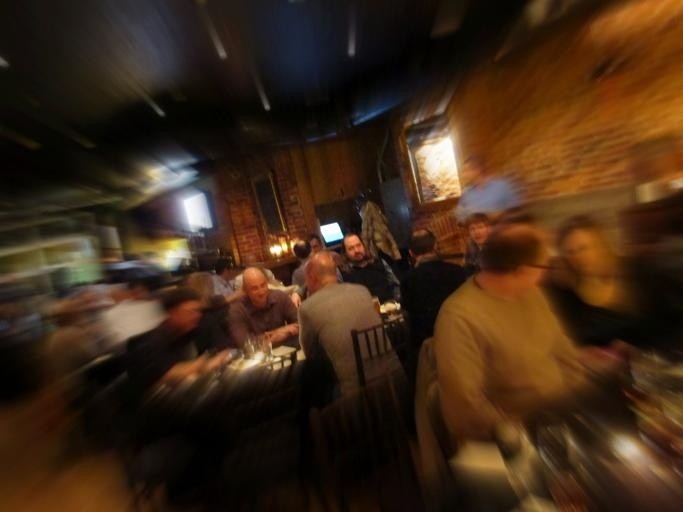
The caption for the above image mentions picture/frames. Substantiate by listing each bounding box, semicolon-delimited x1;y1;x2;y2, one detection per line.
248;170;288;238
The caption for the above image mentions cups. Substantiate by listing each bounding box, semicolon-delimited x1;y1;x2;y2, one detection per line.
242;330;274;363
379;298;402;320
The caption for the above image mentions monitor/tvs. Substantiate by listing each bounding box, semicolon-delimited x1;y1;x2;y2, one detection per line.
320;221;345;245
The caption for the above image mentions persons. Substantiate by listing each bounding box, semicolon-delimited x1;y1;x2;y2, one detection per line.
0;212;465;512
551;212;682;366
460;213;494;266
431;219;619;469
453;156;523;228
